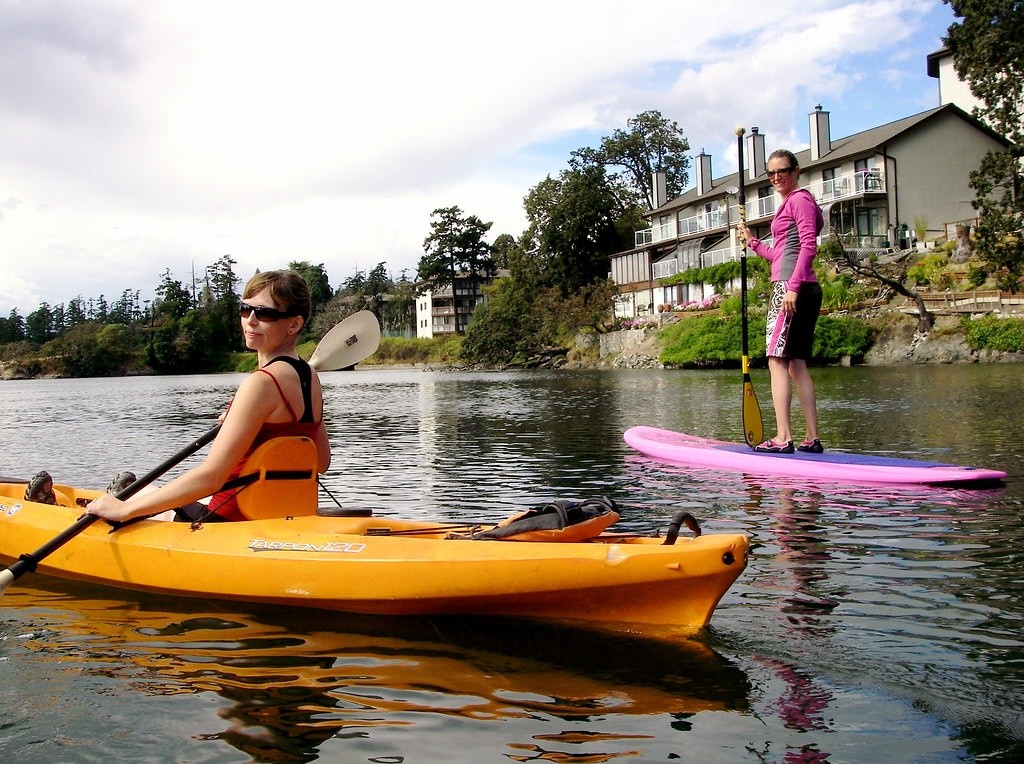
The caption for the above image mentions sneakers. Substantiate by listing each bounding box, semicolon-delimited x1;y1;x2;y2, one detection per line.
23;471;65;506
797;438;824;453
107;471;137;496
752;437;794;454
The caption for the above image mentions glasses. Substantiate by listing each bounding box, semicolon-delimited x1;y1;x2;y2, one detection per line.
239;301;293;322
766;166;792;177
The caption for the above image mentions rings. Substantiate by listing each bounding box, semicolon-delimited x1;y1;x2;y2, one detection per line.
91;498;96;503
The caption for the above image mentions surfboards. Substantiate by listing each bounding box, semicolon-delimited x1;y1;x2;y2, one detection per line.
623;426;1007;482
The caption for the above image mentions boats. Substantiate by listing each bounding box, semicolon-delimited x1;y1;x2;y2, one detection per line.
0;476;752;642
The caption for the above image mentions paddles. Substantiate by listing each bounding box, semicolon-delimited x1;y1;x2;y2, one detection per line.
1;310;381;594
737;128;764;447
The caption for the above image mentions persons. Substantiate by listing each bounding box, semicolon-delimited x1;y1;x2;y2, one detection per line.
23;271;331;523
737;149;824;454
